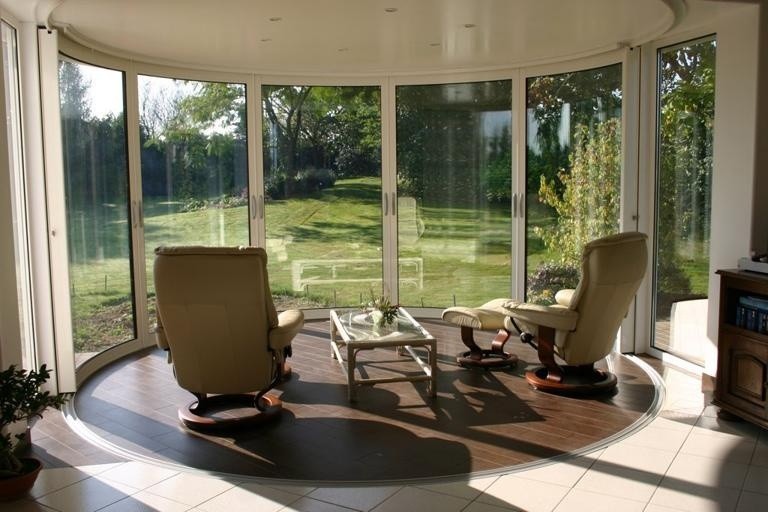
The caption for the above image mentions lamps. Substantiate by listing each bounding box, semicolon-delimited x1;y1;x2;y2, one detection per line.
153;244;304;434
397;197;425;249
502;231;649;398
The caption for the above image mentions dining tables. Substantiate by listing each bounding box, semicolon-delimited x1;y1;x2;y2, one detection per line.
0;365;75;503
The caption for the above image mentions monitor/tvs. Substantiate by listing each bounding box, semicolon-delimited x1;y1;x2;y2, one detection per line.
740;296;767;310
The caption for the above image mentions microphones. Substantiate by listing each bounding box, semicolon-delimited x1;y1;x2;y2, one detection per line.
441;297;518;370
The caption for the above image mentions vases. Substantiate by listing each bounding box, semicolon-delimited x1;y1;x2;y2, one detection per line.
360;295;399;325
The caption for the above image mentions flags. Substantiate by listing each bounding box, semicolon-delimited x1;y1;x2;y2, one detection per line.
368;311;386;329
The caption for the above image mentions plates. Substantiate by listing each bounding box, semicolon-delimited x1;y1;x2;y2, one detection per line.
712;268;768;429
